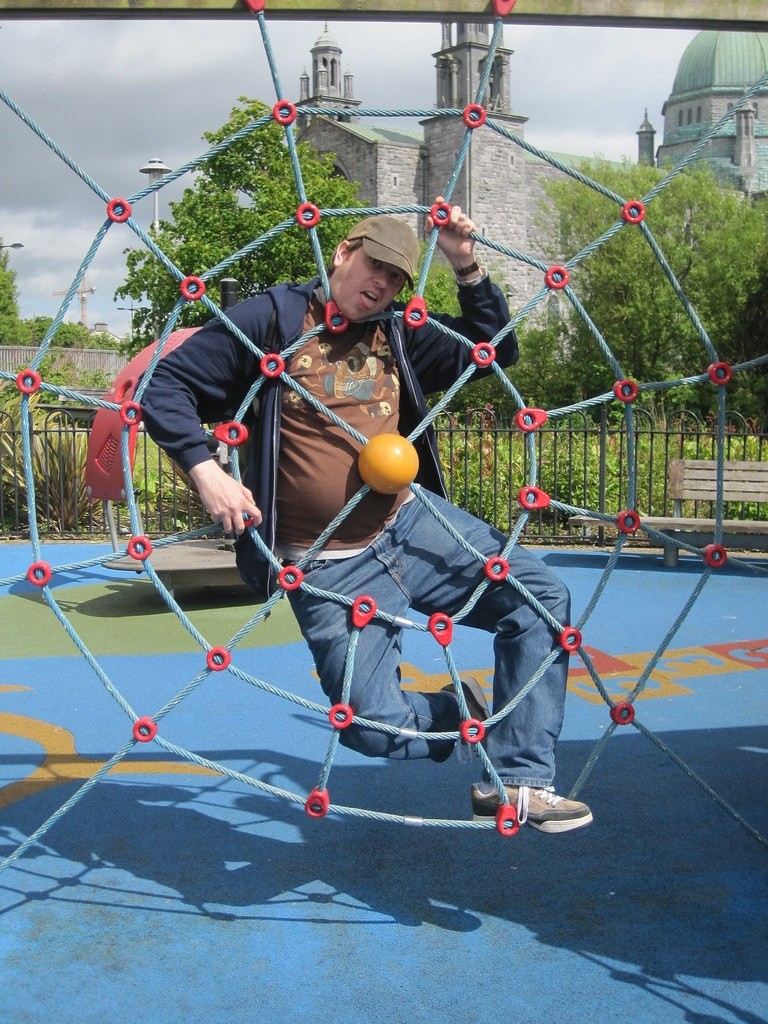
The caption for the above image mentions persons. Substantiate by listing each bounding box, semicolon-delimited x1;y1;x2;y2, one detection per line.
132;197;595;834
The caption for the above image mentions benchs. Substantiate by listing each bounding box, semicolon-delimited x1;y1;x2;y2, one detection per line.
569;460;768;567
19;386;109;421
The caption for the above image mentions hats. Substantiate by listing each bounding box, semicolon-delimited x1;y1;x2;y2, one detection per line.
345;216;420;292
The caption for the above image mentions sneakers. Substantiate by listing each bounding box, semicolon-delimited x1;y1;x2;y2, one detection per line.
471;780;594;835
440;676;493;765
